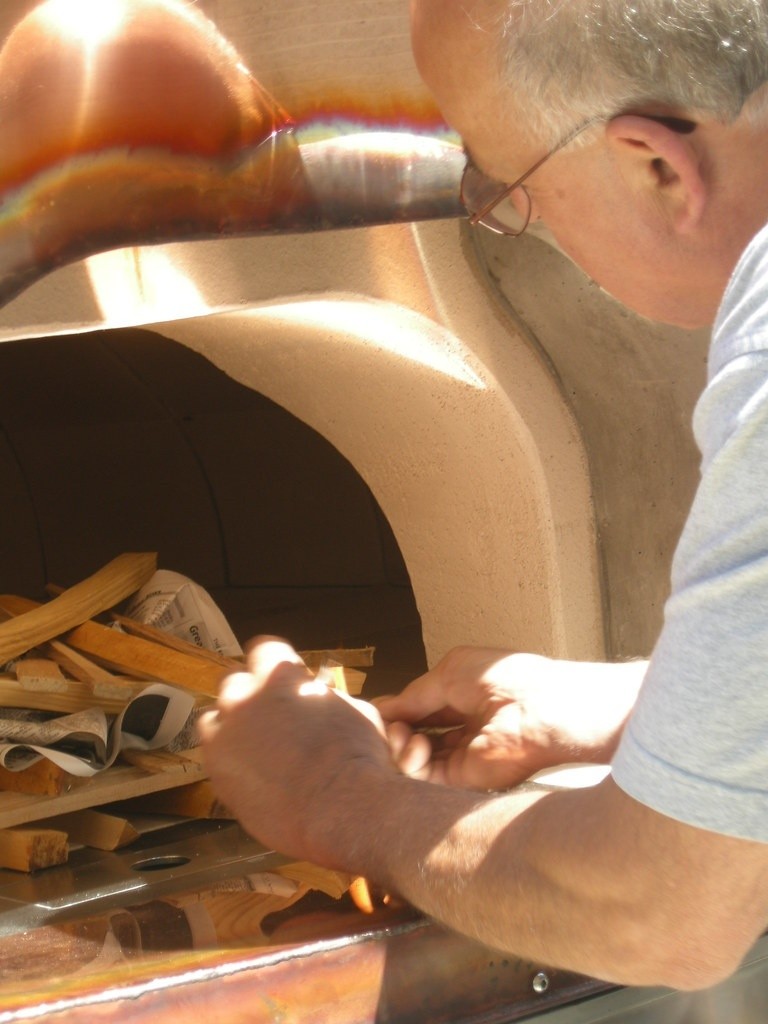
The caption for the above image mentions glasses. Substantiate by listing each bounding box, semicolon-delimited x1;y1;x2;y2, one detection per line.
460;114;698;236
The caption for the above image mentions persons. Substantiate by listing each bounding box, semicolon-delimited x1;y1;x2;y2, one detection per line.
198;0;768;995
